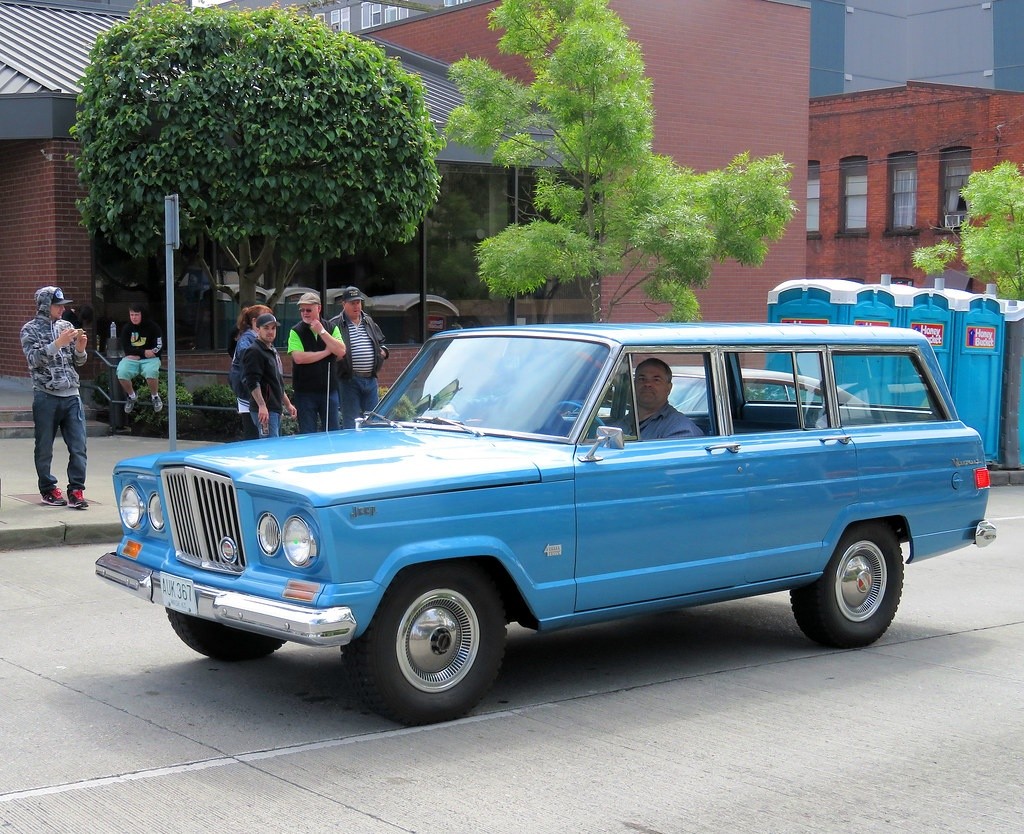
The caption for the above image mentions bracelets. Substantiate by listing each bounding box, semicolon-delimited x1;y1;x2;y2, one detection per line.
319;328;326;336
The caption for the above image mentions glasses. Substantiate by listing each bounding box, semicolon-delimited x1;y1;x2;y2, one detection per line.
636;377;669;384
299;308;318;312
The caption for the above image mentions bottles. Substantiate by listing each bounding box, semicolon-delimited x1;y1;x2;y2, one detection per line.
110;321;117;340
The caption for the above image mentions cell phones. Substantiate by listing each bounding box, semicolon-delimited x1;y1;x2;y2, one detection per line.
75;329;84;338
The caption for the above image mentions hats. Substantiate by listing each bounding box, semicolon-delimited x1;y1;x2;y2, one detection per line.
256;313;281;327
51;289;73;304
297;292;322;305
342;287;364;301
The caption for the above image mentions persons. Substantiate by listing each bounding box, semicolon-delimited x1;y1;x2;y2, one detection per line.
228;302;254;355
243;313;297;438
229;304;272;441
20;286;88;508
328;286;388;431
286;292;346;433
612;358;705;438
116;305;164;412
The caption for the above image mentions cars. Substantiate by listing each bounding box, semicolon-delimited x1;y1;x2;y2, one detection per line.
91;321;993;731
321;288;371;306
261;286;329;306
210;280;277;311
641;363;869;429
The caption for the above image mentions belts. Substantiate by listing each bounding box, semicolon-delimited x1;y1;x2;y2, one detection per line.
354;371;372;378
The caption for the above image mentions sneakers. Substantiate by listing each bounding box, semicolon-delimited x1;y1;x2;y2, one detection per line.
42;489;88;508
125;392;138;413
150;395;164;412
